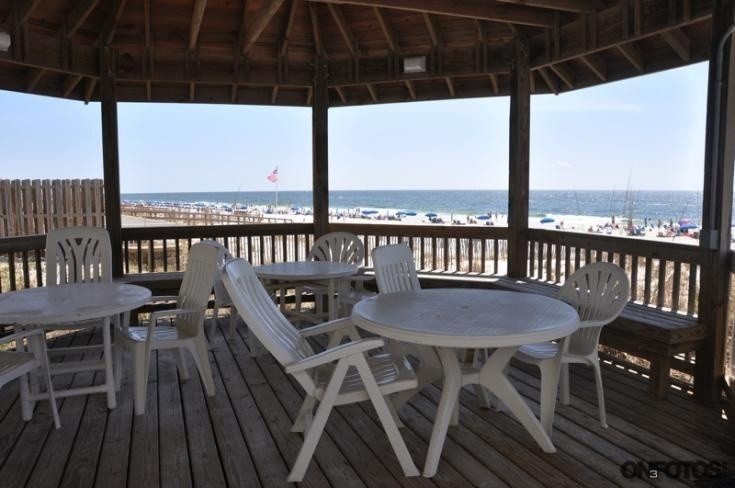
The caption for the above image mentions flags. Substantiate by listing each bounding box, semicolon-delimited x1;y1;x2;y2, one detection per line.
267;169;279;183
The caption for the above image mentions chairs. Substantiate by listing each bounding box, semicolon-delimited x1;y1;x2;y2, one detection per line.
114;238;227;415
279;231;365;327
219;256;422;481
1;327;64;432
15;225;129;389
489;261;629;429
370;243;492;422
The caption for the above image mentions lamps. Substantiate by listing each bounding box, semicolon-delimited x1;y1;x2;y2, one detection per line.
0;31;11;53
404;56;427;74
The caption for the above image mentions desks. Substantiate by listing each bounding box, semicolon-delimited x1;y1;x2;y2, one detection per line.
1;283;152;420
254;259;360;332
350;288;580;477
